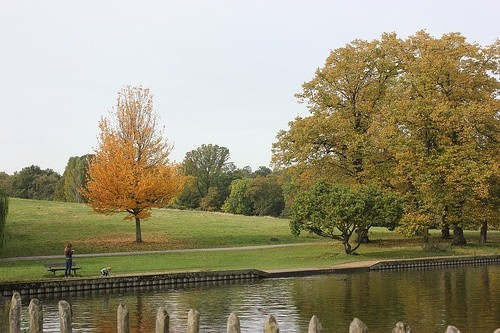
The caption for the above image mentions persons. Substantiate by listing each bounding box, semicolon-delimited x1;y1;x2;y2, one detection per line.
63;242;74;279
101;266;112;277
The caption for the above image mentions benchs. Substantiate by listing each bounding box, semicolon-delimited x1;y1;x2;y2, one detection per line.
43;261;80;275
